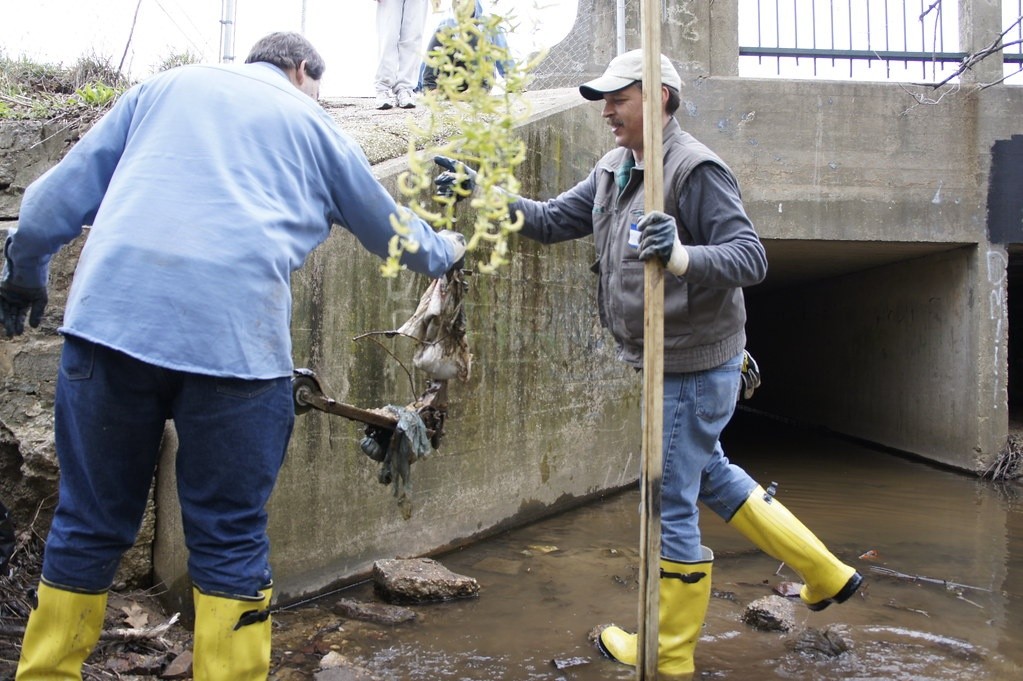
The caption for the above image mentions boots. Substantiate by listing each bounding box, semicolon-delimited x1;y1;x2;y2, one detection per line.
192;579;274;681
16;574;112;681
726;481;865;611
597;545;714;681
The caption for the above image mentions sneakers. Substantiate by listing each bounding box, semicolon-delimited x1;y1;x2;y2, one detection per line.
375;92;393;109
395;88;416;108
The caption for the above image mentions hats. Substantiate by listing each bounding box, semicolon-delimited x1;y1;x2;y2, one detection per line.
579;49;681;101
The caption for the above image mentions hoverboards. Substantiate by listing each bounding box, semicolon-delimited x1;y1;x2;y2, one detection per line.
293;173;473;446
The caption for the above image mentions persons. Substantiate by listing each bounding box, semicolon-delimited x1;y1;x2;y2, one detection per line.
0;30;465;681
374;0;430;108
414;0;528;95
431;49;863;674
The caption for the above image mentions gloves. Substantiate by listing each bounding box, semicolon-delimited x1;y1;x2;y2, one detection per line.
0;281;48;337
438;228;465;271
636;211;689;277
434;155;479;207
740;349;762;400
